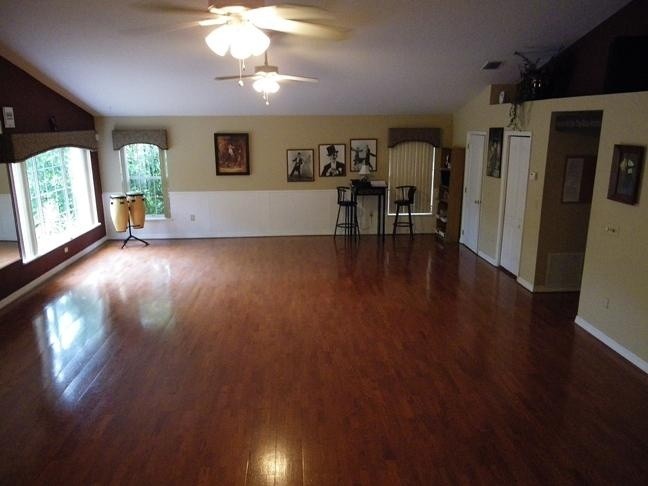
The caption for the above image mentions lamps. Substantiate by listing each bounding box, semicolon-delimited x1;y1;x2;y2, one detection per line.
360;165;371;185
188;0;354;108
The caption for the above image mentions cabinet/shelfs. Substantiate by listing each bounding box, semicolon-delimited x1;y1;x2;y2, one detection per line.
433;147;465;242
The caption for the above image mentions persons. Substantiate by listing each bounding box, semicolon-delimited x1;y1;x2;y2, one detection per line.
290;152;304;177
365;145;376;170
351;147;363;166
321;145;344;176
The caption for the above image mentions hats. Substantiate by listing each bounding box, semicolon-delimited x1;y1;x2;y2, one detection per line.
326;144;339;156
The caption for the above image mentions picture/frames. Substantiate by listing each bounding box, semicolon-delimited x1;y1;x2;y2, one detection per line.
607;144;645;205
287;138;378;182
214;133;249;175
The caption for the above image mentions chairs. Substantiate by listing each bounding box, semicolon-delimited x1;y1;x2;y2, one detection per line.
334;186;417;241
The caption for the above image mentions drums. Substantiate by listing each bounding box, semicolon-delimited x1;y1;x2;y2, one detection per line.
110;195;128;232
126;191;145;228
